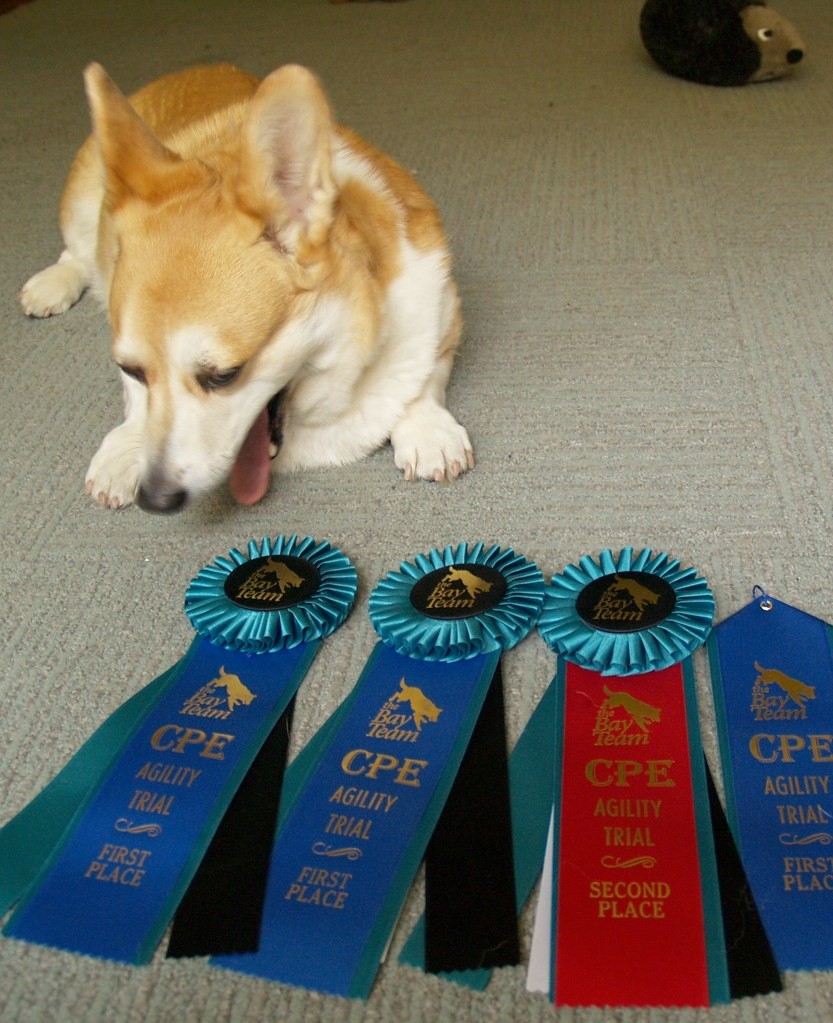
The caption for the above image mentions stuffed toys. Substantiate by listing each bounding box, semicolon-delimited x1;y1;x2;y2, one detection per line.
638;0;805;87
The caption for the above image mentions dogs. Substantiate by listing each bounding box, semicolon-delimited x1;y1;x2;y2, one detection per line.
21;58;474;514
